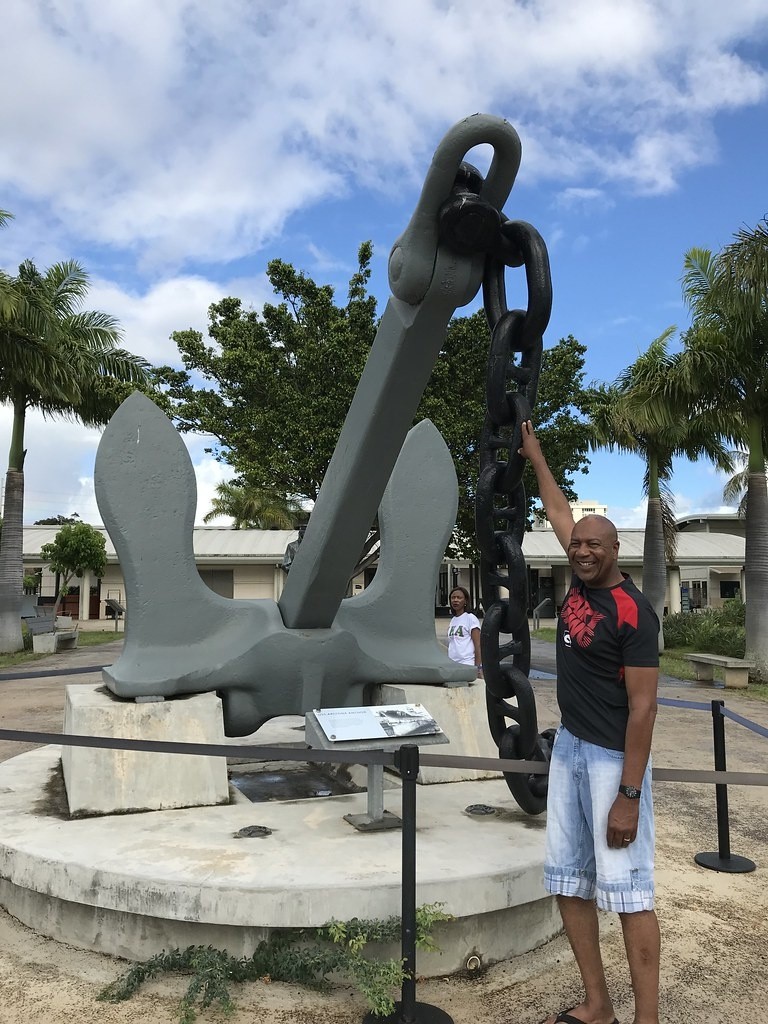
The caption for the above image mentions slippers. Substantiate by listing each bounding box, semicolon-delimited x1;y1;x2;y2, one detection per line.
539;1008;619;1024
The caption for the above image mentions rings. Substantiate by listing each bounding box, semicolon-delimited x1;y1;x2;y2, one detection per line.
624;838;630;841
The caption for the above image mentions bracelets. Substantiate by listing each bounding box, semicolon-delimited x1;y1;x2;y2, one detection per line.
478;666;481;669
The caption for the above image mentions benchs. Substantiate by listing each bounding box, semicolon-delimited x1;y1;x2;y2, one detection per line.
33;629;80;654
683;652;756;689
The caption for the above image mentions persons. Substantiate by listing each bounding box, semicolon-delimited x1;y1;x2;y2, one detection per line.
519;420;661;1024
447;586;483;679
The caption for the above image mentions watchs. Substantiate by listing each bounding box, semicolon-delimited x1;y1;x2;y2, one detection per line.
619;785;641;798
